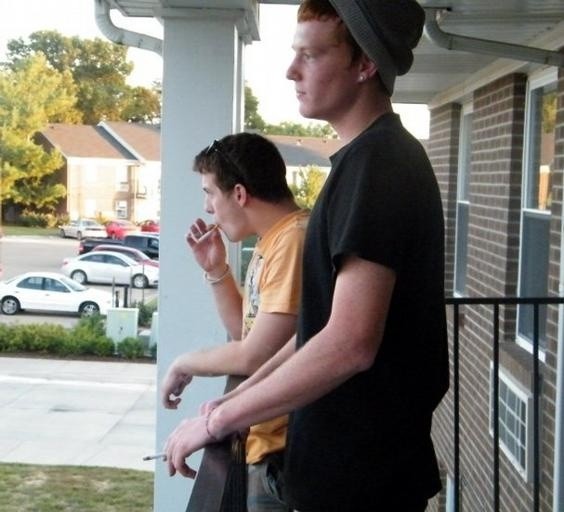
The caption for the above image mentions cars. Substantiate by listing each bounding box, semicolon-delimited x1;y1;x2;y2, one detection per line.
58;211;160;288
0;272;123;324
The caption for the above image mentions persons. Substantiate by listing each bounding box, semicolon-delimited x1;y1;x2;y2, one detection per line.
159;130;312;511
162;2;450;509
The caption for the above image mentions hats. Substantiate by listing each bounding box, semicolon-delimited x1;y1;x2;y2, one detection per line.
326;1;424;94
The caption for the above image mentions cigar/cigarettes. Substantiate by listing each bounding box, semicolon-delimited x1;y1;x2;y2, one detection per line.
143;452;171;463
195;222;219;245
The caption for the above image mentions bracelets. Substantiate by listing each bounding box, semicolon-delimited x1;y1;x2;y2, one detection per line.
203;261;231;288
204;404;220;442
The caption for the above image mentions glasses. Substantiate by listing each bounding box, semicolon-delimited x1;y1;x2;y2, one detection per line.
204;139;245;181
